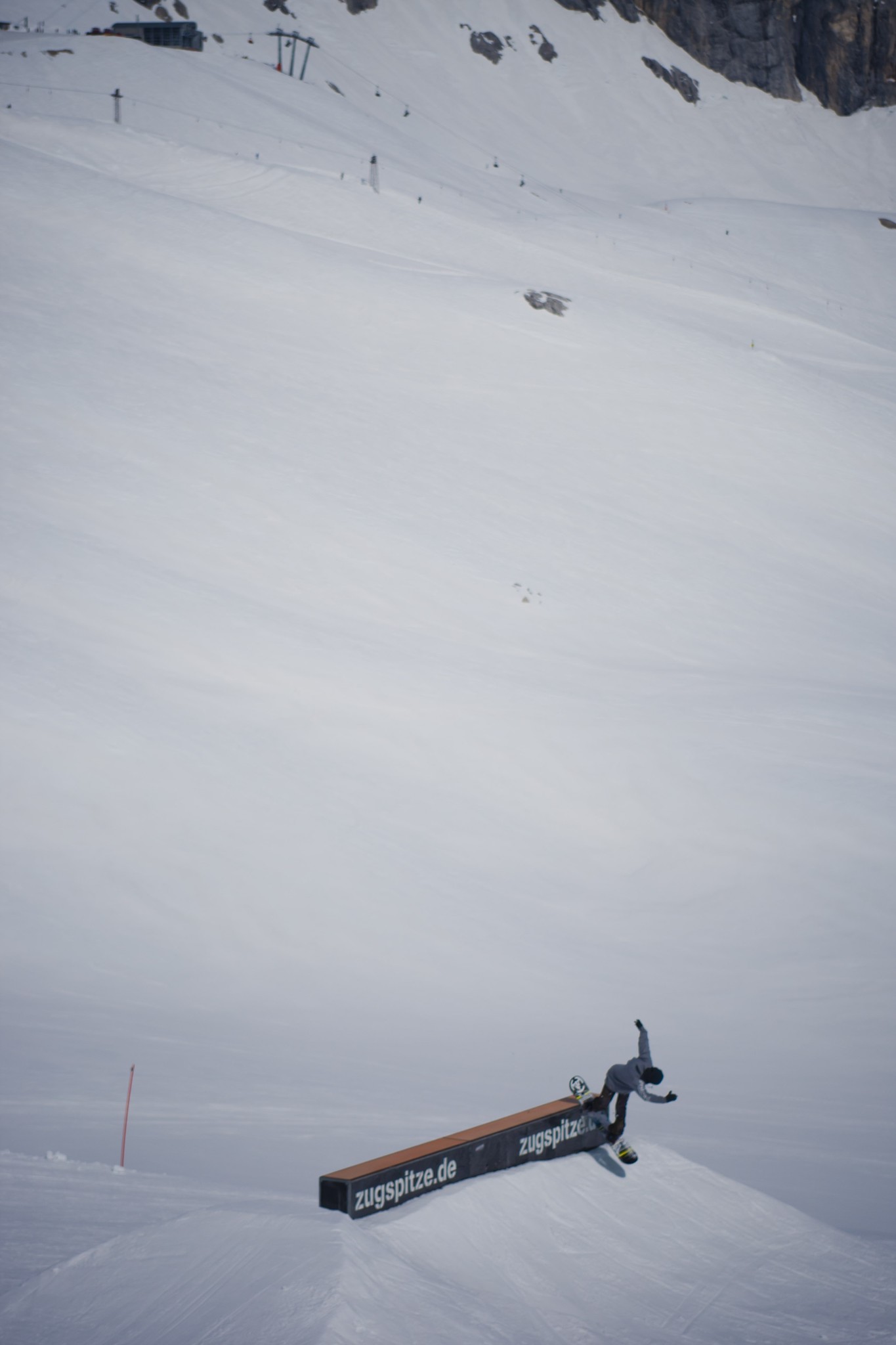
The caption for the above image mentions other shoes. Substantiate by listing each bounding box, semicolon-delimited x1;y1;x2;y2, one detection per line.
583;1100;599;1111
607;1124;618;1143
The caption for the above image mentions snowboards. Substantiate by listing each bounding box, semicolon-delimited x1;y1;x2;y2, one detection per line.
569;1075;638;1166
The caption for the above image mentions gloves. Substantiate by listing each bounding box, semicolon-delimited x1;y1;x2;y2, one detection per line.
666;1091;676;1102
634;1019;642;1028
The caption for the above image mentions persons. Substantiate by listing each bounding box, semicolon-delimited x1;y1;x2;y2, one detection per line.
585;1019;677;1138
